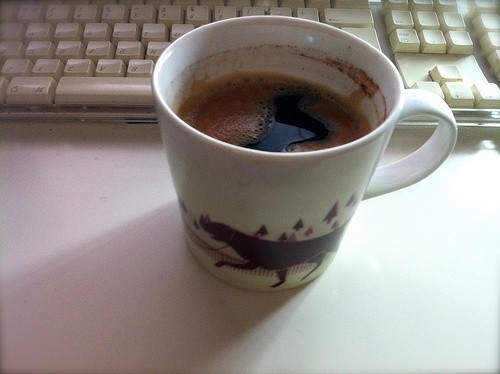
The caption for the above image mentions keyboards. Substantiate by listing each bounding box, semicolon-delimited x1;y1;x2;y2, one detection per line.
0;0;500;132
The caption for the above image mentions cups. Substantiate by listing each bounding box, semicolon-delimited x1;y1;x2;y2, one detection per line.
152;14;457;293
